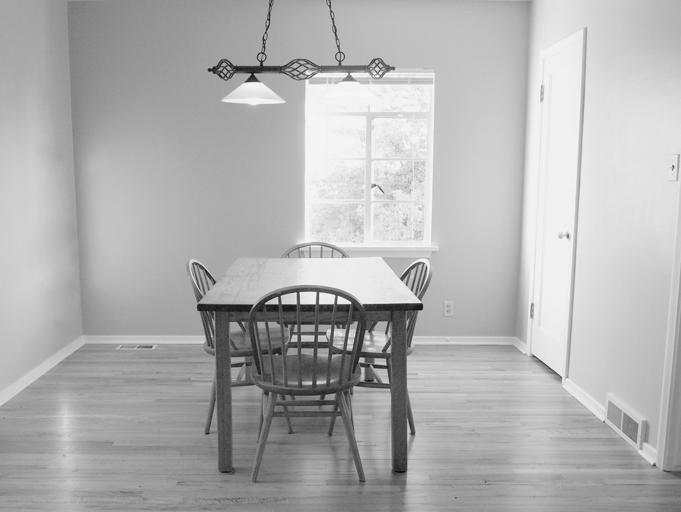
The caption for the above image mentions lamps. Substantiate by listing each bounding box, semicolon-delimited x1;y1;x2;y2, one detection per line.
208;1;397;107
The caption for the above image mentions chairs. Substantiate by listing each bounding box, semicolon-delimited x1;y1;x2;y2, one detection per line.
243;282;369;483
318;255;431;439
187;257;297;438
272;240;362;409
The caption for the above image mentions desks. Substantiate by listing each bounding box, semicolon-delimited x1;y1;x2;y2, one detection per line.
194;254;427;478
